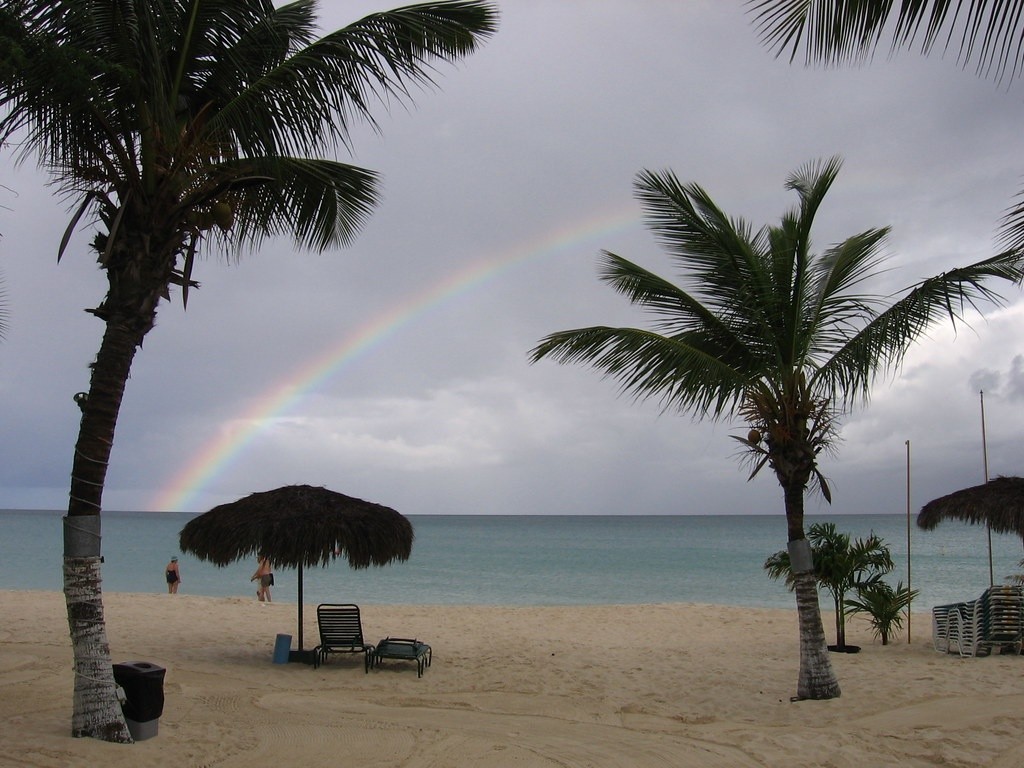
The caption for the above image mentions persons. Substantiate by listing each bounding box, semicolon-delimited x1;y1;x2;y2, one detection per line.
251;551;274;602
166;556;180;593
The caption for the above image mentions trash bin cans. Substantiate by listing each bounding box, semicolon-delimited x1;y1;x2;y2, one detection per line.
112;661;166;742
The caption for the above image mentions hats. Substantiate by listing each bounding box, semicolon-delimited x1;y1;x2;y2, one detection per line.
171;556;178;561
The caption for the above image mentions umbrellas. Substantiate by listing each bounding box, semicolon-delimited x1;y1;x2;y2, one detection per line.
180;484;415;652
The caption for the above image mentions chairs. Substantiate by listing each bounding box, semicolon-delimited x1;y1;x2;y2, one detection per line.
310;601;373;673
369;635;433;678
931;584;1022;658
